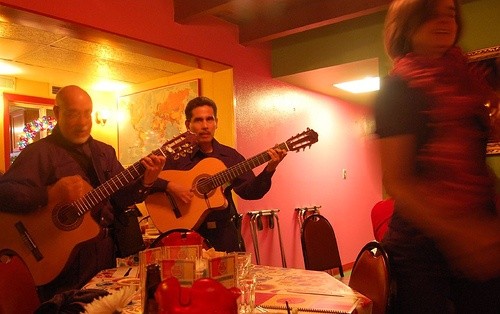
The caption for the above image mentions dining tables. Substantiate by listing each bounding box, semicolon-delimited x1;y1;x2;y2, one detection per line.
85;262;364;313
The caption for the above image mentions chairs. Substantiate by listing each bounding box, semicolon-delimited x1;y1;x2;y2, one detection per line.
300;215;352;285
347;239;397;314
0;248;39;314
150;229;207;251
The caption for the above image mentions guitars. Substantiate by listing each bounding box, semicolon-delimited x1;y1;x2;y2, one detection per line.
0;127;200;289
144;127;319;236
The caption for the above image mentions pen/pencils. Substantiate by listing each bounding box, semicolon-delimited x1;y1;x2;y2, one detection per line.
125;267;133;276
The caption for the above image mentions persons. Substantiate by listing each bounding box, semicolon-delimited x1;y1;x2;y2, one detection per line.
150;96;287;254
374;0;500;313
0;85;166;299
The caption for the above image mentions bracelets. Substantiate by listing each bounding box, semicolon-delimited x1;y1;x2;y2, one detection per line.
144;183;154;187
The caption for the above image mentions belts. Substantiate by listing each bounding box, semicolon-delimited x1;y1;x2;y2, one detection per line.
201;219;232;229
